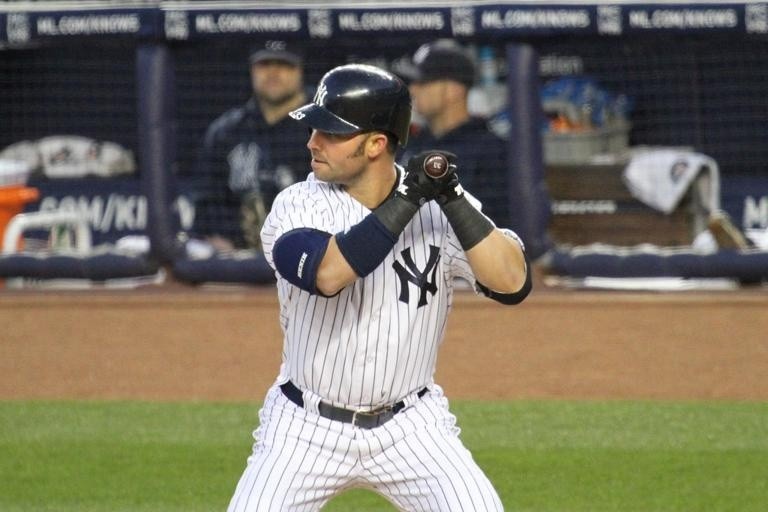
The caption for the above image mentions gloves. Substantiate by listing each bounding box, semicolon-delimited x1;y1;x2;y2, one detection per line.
396;150;465;204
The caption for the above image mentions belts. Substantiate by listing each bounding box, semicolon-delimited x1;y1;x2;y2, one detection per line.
275;378;435;430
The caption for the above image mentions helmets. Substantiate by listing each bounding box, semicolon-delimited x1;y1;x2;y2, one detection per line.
286;63;413;148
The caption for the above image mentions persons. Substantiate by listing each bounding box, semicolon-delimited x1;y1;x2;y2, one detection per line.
391;40;514;238
227;63;530;511
193;35;321;266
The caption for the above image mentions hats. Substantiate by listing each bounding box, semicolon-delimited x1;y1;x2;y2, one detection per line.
250;35;301;66
389;44;474;87
